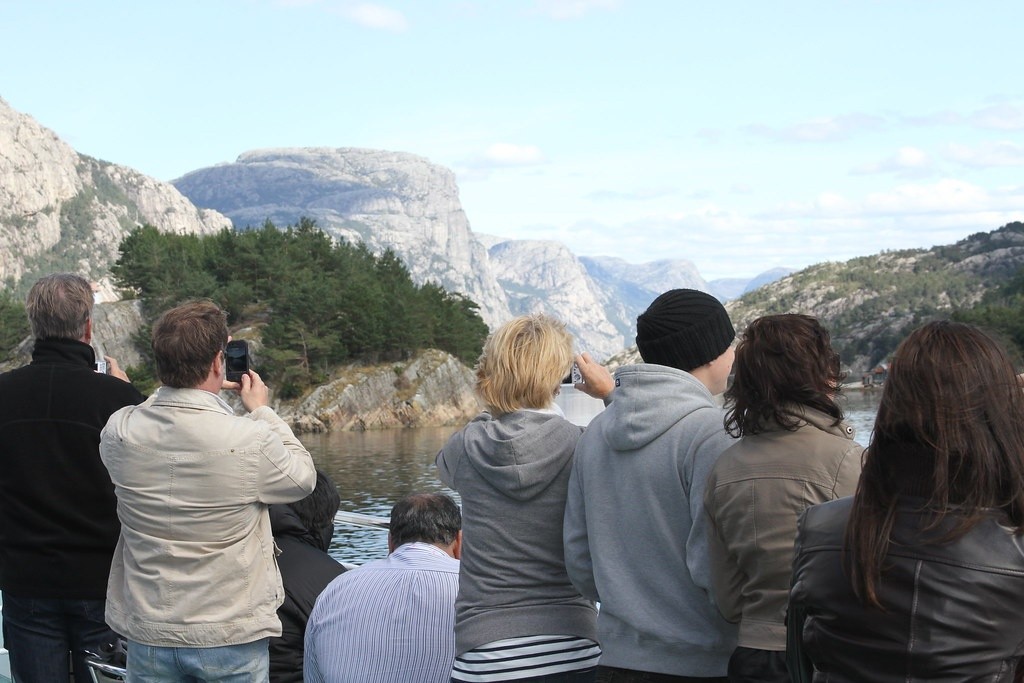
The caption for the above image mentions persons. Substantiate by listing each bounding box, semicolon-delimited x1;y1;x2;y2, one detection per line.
1;274;1024;683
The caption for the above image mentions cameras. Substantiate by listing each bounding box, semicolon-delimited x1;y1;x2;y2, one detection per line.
559;361;583;387
93;361;107;374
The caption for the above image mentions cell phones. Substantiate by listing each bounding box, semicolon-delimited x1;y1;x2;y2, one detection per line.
225;339;250;383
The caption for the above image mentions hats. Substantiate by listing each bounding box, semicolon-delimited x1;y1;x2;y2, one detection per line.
636;289;736;372
